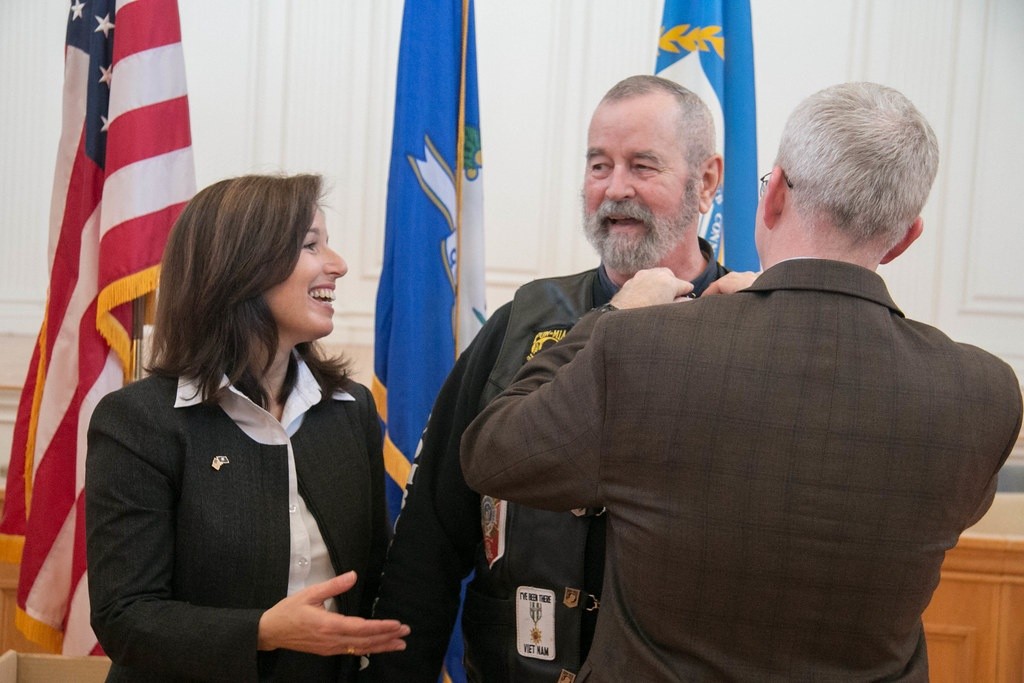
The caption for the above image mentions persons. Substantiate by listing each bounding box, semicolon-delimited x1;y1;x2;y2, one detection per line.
459;82;1023;683
85;174;410;683
370;76;731;682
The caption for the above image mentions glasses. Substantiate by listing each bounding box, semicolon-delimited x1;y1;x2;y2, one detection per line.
760;166;793;192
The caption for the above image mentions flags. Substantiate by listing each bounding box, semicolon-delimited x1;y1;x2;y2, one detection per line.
371;0;483;682
654;0;759;273
0;1;196;657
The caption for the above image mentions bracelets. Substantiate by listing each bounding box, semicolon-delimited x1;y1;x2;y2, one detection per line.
596;303;617;313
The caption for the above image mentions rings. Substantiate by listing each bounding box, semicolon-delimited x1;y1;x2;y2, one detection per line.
348;647;355;653
